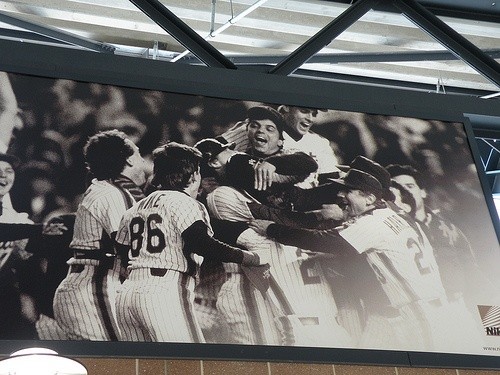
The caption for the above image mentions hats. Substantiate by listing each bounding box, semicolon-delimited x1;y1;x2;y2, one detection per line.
325;170;383;199
250;105;286;139
386;164;427;186
335;156;389;189
197;139;236;164
0;153;24;179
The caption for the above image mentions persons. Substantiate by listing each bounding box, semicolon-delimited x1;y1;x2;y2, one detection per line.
0;72;500;350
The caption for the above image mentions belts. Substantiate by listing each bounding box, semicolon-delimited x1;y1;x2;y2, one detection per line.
296;316;320;325
150;269;166;276
70;266;84;274
